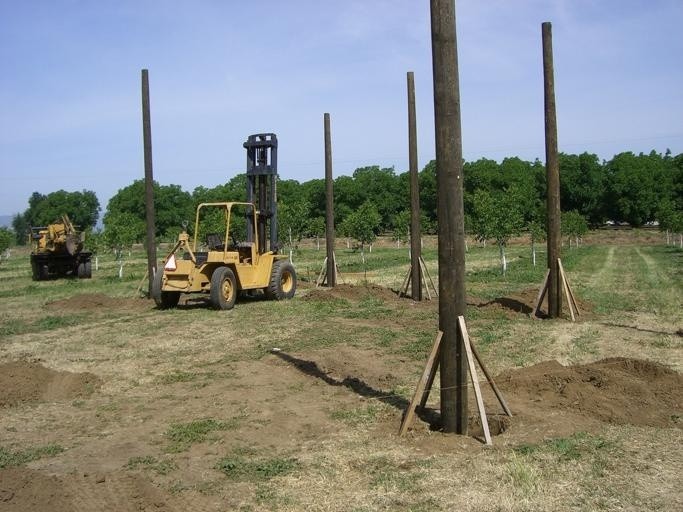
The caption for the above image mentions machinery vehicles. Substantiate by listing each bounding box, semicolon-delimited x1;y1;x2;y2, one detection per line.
28;212;91;280
150;131;295;310
